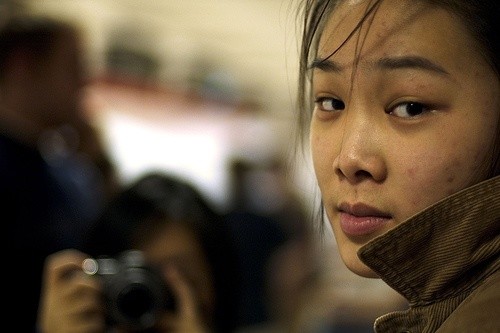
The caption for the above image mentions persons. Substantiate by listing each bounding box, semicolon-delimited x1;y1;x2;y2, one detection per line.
291;0;499;331
1;15;123;330
40;173;259;332
218;157;312;331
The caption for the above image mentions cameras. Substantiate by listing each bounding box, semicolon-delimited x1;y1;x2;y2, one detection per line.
79;253;180;333
